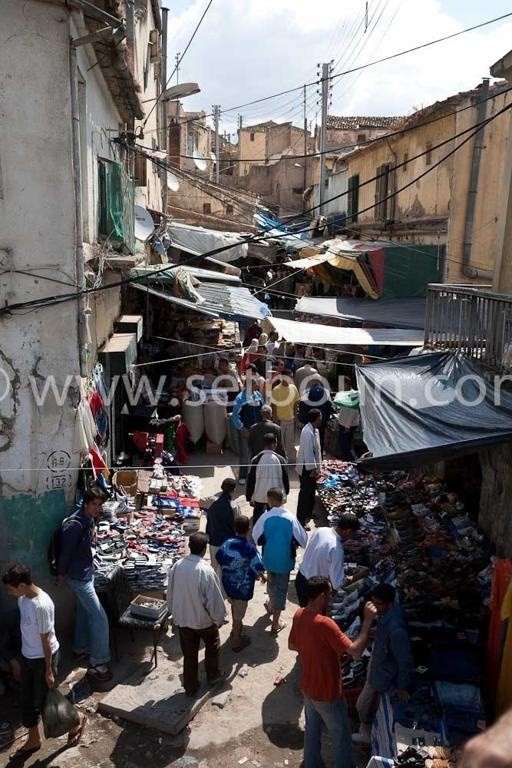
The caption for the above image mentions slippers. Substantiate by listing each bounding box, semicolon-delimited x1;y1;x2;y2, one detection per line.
9;741;41;763
68;715;87;748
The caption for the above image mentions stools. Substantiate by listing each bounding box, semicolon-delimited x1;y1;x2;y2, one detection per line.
118;598;171;670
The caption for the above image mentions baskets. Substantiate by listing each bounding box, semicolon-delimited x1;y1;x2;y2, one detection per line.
130;594;168;620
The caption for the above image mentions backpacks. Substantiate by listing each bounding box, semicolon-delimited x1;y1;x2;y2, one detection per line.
48;512;85;576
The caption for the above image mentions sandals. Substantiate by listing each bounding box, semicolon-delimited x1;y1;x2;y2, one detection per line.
266;597;287;634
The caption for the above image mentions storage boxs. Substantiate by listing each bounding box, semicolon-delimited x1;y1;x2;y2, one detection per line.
112;470;139;495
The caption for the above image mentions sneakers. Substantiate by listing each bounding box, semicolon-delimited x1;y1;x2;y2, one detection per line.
85;664;113;682
351;732;372;744
206;670;231;685
231;635;250;652
186;681;203;697
